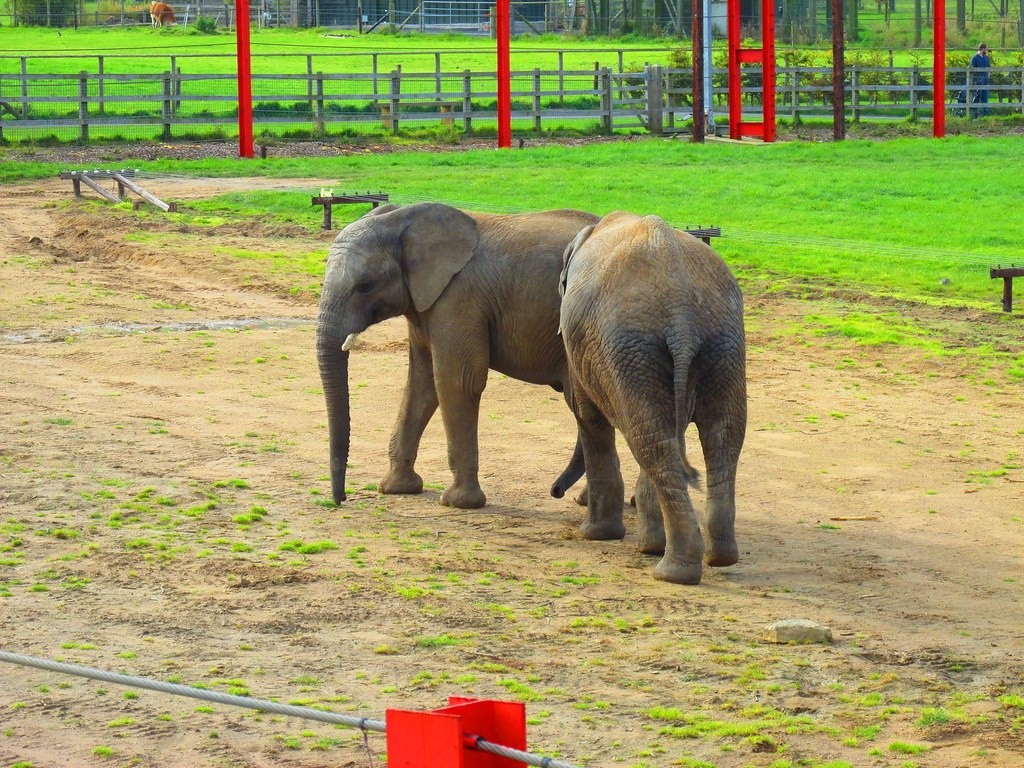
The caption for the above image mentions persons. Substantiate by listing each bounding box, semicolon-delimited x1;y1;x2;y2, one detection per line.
972;43;990;116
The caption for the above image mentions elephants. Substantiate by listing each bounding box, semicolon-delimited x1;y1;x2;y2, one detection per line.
314;202;748;586
